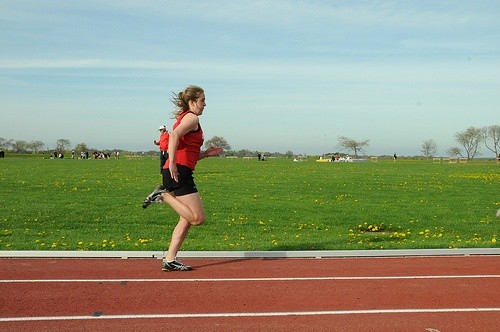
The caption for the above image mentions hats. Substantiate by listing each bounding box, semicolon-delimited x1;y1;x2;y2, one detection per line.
158;125;166;131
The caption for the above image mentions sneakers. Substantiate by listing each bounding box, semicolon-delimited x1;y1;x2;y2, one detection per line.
162;258;192;271
142;184;168;209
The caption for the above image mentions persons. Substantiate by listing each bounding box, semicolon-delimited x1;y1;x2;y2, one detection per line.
153;125;171;175
0;148;500;162
142;85;223;272
